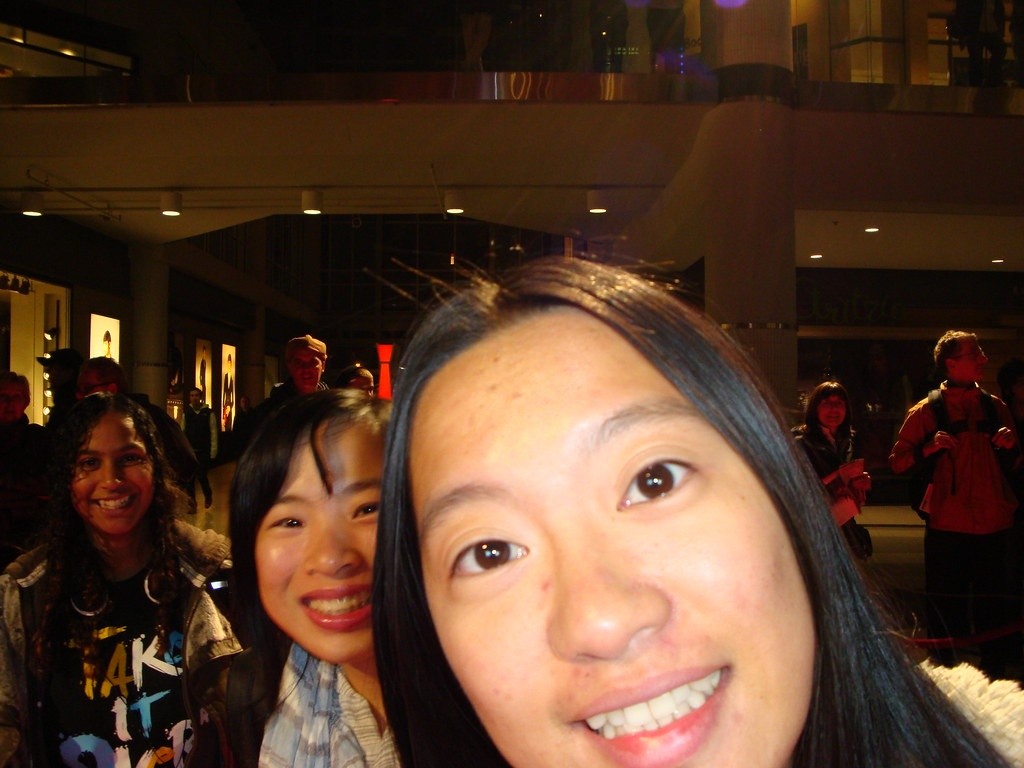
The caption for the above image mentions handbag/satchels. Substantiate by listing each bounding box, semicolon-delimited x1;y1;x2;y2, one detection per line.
829;485;860;527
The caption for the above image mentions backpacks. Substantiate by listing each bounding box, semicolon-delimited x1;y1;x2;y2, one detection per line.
895;390;1007;522
144;405;198;484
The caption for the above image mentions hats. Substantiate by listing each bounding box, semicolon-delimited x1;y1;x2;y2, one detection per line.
285;335;326;361
36;348;82;377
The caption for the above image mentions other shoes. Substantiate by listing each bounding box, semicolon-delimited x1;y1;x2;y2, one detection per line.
188;508;196;514
205;498;212;508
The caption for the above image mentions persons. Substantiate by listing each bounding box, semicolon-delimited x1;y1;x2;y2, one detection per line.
1;389;243;768
0;335;375;570
791;378;873;559
366;259;1023;768
885;330;1024;683
187;386;403;768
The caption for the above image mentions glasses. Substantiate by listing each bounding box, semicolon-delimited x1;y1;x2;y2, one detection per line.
77;381;119;393
819;399;844;407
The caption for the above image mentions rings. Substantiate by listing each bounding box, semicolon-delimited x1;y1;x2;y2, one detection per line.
1007;435;1011;441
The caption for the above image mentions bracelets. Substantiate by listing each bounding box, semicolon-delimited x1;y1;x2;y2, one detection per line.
912;444;924;462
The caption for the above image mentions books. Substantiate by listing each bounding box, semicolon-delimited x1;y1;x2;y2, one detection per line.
820;458;867;527
